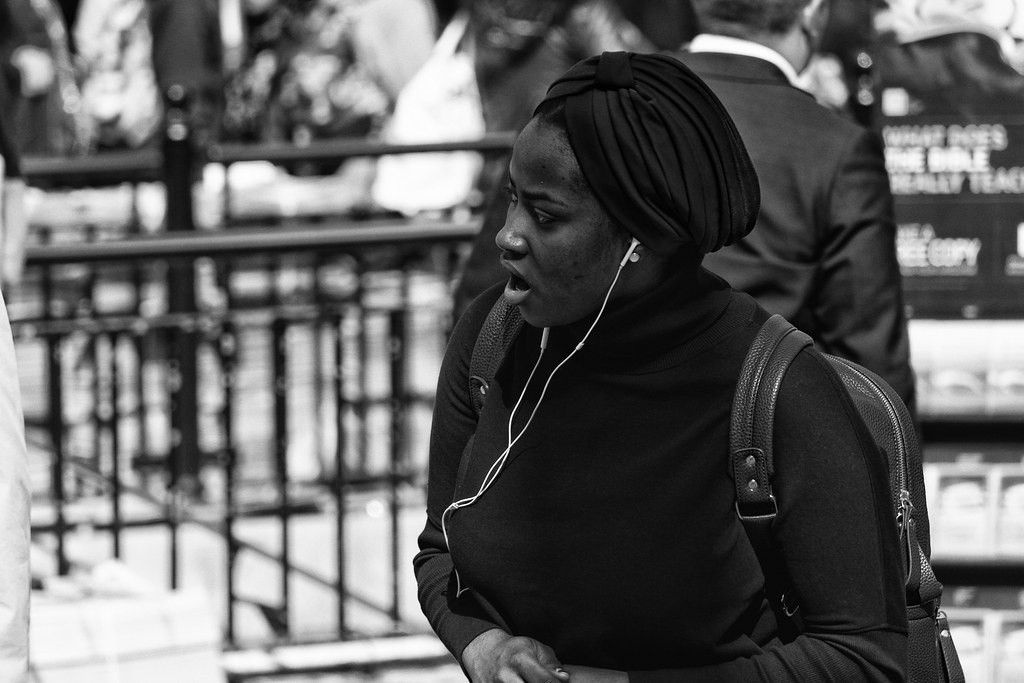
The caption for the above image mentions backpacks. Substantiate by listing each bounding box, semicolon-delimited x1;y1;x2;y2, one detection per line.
470;278;965;683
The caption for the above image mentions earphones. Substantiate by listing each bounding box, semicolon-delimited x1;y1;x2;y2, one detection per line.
620;237;641;270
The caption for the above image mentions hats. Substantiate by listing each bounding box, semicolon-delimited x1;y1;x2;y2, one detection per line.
532;46;760;253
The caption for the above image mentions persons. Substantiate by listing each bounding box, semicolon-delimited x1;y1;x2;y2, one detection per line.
671;0;918;426
411;47;908;683
